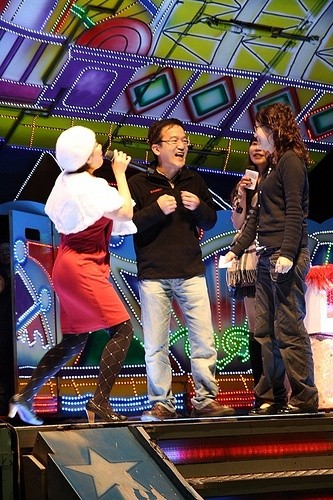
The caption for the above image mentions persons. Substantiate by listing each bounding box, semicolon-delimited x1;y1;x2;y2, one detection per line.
226;133;296;409
224;102;319;415
8;126;133;425
127;118;218;421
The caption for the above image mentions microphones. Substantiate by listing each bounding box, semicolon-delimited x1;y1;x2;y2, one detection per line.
104;150;147;173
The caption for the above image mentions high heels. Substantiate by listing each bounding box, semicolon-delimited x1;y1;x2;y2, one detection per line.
8;392;44;425
83;398;127;423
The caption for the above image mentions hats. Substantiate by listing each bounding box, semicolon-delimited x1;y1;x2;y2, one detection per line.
56;126;96;172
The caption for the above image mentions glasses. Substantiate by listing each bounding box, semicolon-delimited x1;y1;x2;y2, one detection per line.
253;125;263;131
156;138;190;146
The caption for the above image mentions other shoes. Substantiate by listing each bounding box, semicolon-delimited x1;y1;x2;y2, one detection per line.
278;403;317;414
248;402;283;415
191;400;236;416
140;402;179;420
